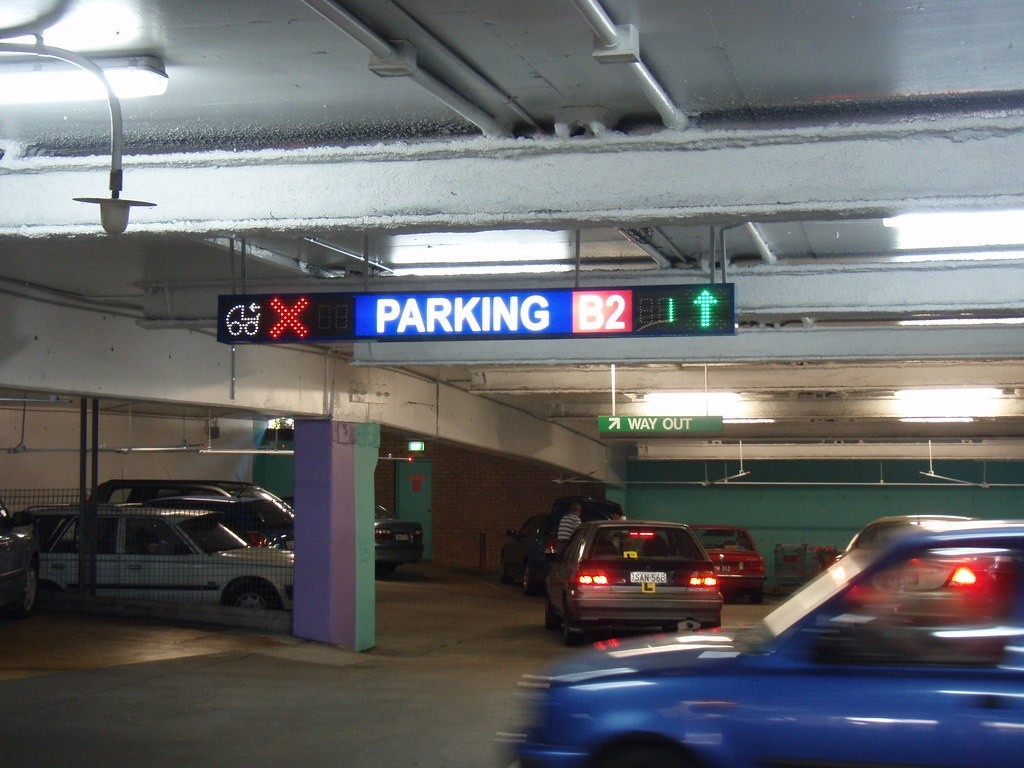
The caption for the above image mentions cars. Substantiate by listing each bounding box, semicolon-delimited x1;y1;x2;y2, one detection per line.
687;525;767;607
374;505;424;573
822;512;1012;662
501;494;625;596
540;520;724;647
1;499;41;621
11;479;296;612
508;523;1024;768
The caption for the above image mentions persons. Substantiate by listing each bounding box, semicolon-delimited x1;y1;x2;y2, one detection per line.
558;503;581;556
609;503;628;547
